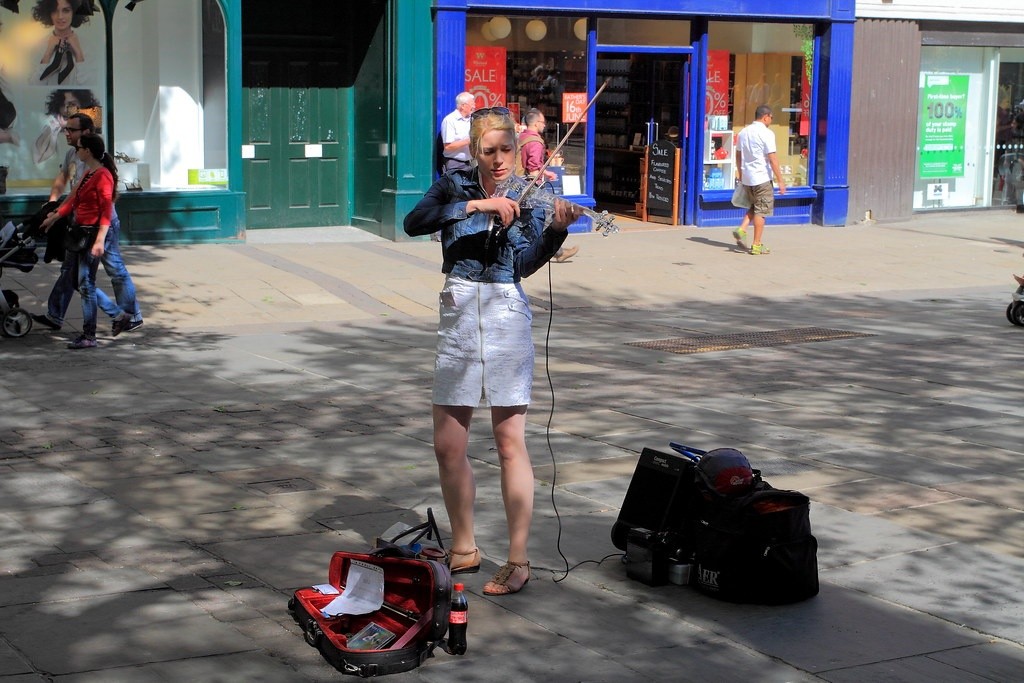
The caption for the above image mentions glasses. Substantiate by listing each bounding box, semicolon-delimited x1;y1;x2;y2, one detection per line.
470;106;511;129
77;146;85;151
65;127;82;132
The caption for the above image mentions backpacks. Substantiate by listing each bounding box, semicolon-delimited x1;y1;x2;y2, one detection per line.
515;136;545;177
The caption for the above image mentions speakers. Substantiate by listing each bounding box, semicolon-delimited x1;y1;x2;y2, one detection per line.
611;447;699;558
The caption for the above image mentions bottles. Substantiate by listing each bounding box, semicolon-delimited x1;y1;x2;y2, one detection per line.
447;583;468;655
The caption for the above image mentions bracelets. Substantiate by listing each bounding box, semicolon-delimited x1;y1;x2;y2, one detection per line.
53;210;57;213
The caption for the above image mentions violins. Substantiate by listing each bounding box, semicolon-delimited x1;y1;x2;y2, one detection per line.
492;174;619;237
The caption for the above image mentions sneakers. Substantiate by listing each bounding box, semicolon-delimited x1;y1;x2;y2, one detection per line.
750;243;771;255
112;314;132;336
126;320;143;331
31;313;61;330
733;228;749;249
556;246;579;263
68;333;97;348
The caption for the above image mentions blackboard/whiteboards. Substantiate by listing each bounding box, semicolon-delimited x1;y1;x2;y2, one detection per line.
643;141;680;217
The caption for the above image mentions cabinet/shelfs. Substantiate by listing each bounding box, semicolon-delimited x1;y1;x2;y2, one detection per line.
704;130;734;191
506;49;657;148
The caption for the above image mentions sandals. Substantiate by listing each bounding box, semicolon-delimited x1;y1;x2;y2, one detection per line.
483;560;531;596
447;548;481;574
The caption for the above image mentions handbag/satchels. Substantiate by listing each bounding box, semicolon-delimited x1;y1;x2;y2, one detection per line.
731;182;751;209
65;224;92;253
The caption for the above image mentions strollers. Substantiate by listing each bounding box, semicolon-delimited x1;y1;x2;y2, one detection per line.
0;201;59;338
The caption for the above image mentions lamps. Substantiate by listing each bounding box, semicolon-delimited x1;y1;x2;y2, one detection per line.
525;18;547;41
574;17;587;41
480;15;511;42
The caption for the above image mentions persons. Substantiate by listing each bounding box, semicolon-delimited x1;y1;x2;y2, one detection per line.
441;92;475;171
732;105;786;255
33;1;103;180
402;111;583;596
30;113;143;349
518;111;579;262
